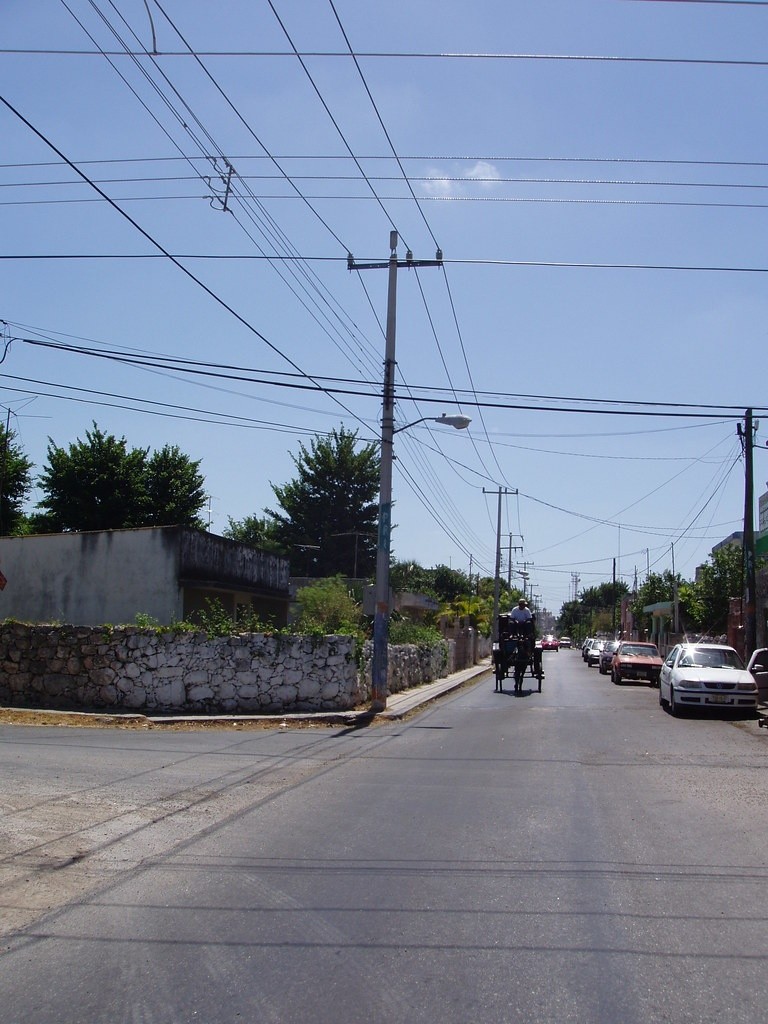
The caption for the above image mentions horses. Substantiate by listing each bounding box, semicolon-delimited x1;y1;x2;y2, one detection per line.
514;636;537;695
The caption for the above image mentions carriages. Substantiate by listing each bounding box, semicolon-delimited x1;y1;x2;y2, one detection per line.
490;614;546;694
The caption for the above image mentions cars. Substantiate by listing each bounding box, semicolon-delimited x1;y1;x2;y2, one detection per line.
597;641;633;675
658;643;768;716
581;638;610;667
611;642;665;685
559;637;571;648
541;637;559;652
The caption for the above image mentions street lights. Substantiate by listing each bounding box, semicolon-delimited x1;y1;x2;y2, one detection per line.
490;569;530;663
370;414;472;713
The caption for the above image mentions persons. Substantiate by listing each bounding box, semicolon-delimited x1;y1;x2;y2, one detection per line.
508;598;534;640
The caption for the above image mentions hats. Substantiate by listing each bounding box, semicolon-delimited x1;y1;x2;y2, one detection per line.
518;599;528;604
